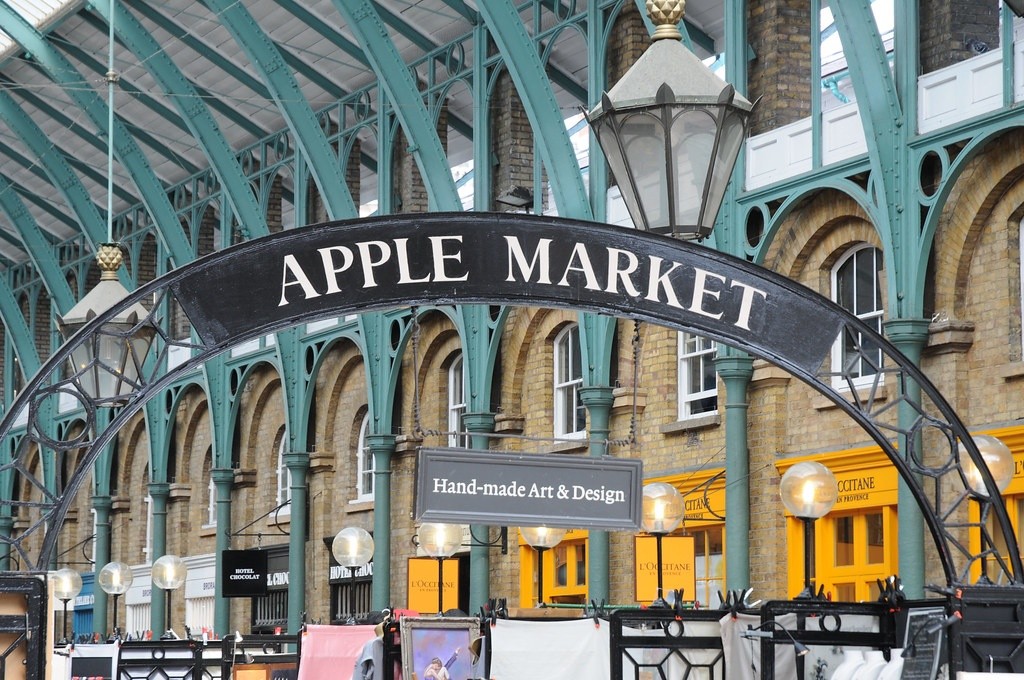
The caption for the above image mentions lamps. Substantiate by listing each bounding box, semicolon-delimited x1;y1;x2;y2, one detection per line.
232;630;243;665
53;0;164;410
641;482;687;608
519;526;566;607
53;568;83;645
99;561;134;644
245;653;255;664
153;554;186;640
494;185;544;214
948;435;1013;586
579;0;765;243
779;459;838;602
331;527;376;626
419;523;463;617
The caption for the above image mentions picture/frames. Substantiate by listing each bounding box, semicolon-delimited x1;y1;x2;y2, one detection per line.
400;616;481;680
